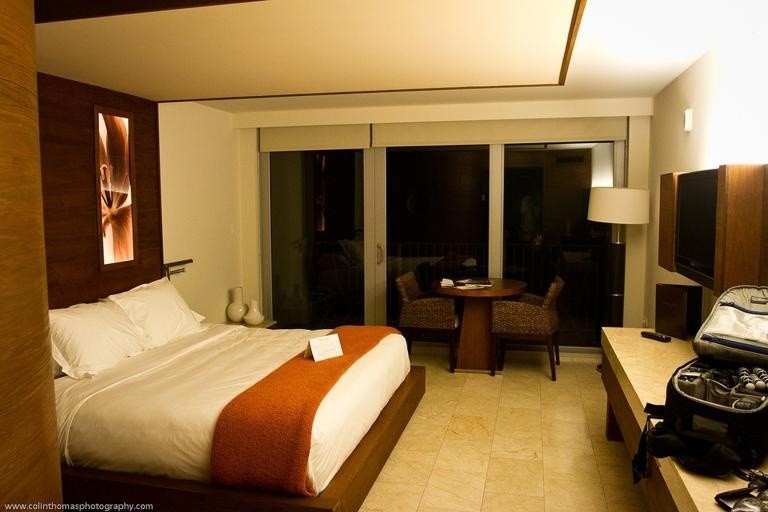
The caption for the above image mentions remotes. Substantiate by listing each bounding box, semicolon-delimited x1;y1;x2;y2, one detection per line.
641;332;671;342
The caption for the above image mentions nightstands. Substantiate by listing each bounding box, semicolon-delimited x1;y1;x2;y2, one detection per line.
223;319;279;330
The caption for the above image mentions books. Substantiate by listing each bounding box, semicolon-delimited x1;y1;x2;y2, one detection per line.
454;278;495;290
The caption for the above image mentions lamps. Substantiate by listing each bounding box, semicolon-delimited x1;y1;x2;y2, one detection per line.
583;186;653;374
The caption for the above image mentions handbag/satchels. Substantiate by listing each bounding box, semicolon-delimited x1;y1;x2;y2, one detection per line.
664;284;767;470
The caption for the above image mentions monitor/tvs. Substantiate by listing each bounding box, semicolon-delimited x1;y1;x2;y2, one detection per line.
675;170;718;278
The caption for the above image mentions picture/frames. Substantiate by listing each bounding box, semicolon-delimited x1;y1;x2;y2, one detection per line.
91;106;140;273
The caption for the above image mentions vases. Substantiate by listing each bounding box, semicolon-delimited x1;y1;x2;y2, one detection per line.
225;286;248;323
241;299;265;325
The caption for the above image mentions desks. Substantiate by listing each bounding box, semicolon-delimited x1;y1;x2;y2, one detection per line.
431;277;528;372
598;324;768;512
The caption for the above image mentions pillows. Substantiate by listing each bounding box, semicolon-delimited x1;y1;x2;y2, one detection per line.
108;276;207;351
47;297;150;382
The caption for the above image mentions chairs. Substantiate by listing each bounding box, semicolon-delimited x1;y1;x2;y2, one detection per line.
486;274;565;383
394;270;461;373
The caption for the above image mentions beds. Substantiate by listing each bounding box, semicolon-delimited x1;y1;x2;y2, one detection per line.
51;321;428;512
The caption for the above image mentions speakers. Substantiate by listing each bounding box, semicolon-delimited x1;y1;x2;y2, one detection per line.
655;283;703;340
